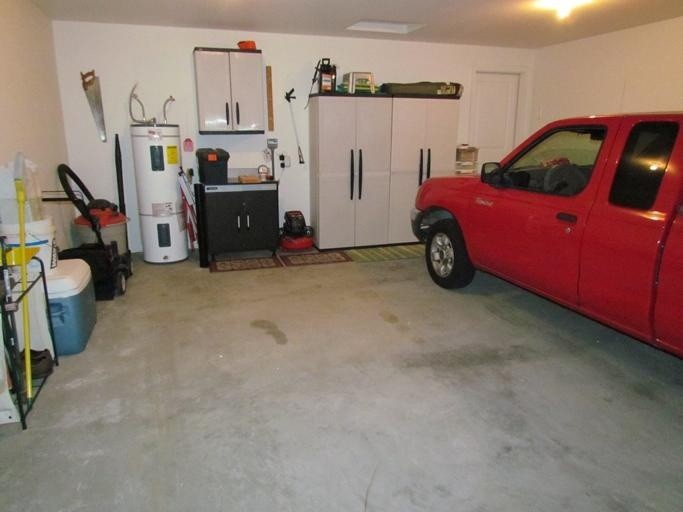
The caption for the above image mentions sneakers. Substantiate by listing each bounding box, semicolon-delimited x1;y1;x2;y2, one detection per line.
19;348;53;378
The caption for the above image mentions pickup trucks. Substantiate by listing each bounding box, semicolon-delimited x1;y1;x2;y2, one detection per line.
410;112;683;360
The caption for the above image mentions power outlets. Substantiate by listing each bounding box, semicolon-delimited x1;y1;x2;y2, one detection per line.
282;156;290;166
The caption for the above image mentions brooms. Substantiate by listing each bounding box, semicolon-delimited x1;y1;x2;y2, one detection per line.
14;151;33;407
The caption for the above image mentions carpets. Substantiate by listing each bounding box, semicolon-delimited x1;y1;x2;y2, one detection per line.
208;245;353;273
344;243;426;263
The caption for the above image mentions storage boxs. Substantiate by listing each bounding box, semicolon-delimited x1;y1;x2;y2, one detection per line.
196;147;230;184
44;259;96;355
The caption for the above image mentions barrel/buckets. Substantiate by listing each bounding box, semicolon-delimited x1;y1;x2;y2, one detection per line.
456;144;478;174
0;215;58;275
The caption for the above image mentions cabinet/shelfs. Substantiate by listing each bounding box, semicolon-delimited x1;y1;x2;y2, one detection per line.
0;256;59;431
192;47;265;135
194;182;279;270
309;94;393;252
389;94;460;245
